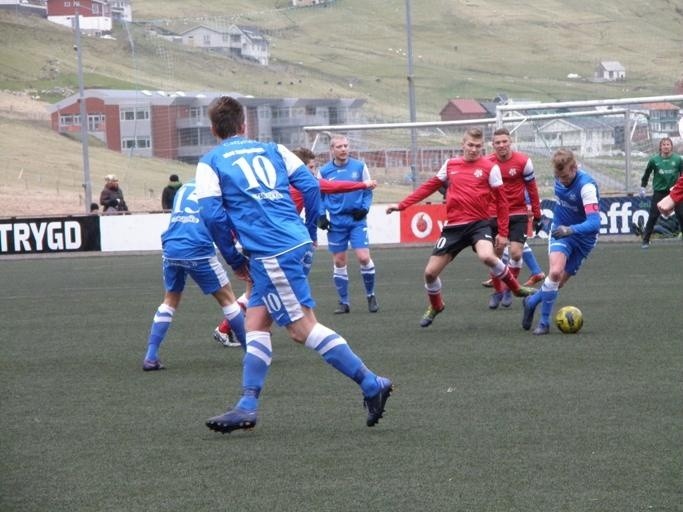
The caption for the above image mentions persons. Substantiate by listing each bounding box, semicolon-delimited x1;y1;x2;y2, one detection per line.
657;173;683;216
214;147;376;348
143;179;246;371
317;134;378;314
482;129;546;309
386;128;540;327
196;98;394;434
641;138;683;249
523;150;601;335
89;175;132;216
481;187;545;288
162;175;183;213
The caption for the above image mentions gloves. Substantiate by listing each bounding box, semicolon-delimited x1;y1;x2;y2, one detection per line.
531;217;542;235
352;208;367;221
551;226;572;240
640;188;646;200
319;216;332;232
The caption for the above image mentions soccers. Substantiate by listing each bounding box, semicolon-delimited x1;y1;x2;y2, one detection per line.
557;306;583;333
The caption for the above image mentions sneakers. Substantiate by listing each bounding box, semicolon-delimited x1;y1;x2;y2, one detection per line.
204;409;257;434
640;241;649;249
481;279;493;287
533;321;549;334
143;358;166;371
420;303;445;327
521;296;534;329
362;376;393;427
367;294;378;312
515;287;538;296
212;326;241;347
502;290;512;306
333;300;349;314
488;292;502;308
522;272;545;286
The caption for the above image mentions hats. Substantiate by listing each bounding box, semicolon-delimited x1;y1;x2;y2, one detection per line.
169;175;178;181
